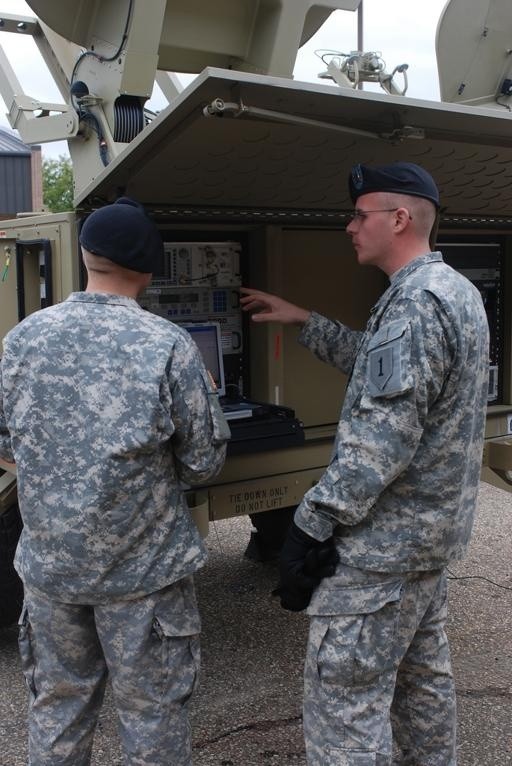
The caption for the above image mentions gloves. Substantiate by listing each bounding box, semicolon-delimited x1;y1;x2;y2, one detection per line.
271;526;339;611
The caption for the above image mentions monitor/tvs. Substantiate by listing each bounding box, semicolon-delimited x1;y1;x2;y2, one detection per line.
176;320;227;398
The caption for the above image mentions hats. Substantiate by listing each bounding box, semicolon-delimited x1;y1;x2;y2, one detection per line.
348;162;440;208
79;197;164;273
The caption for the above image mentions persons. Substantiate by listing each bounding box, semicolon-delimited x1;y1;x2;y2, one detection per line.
0;203;230;766
238;161;490;766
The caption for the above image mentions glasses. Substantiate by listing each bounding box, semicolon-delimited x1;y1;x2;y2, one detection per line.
350;209;413;220
